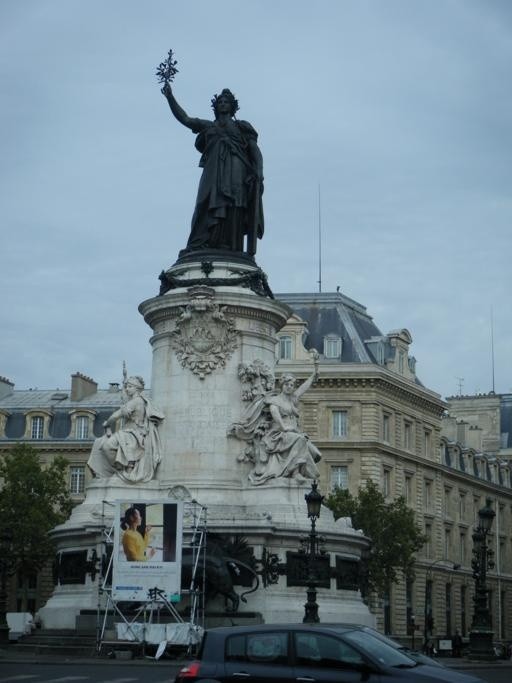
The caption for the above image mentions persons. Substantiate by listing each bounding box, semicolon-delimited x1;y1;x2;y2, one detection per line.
87;360;166;484
120;508;155;561
161;83;263;256
226;371;322;481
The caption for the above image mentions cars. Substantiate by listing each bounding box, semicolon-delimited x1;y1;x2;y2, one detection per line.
170;617;486;683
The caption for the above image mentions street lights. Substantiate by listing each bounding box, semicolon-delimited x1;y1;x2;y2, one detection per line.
303;475;326;624
465;499;502;665
